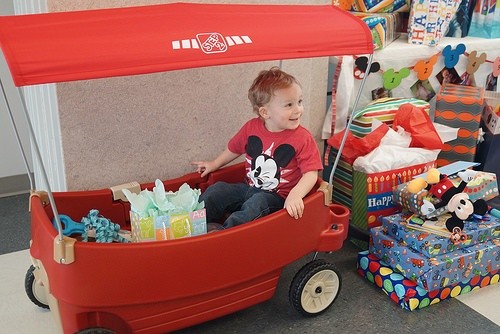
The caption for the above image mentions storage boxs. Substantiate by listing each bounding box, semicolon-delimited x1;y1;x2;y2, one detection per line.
320;84;500;312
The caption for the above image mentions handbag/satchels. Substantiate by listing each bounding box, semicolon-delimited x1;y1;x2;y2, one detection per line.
122;180;208;243
327;103;448;174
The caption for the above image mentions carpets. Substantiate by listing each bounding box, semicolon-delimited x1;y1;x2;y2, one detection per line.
73;239;500;333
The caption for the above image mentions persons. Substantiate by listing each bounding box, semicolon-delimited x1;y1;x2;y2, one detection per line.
416;81;434;102
190;67;324;230
441;68;459;85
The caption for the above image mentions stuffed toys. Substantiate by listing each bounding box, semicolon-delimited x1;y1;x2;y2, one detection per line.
407;168;487;232
